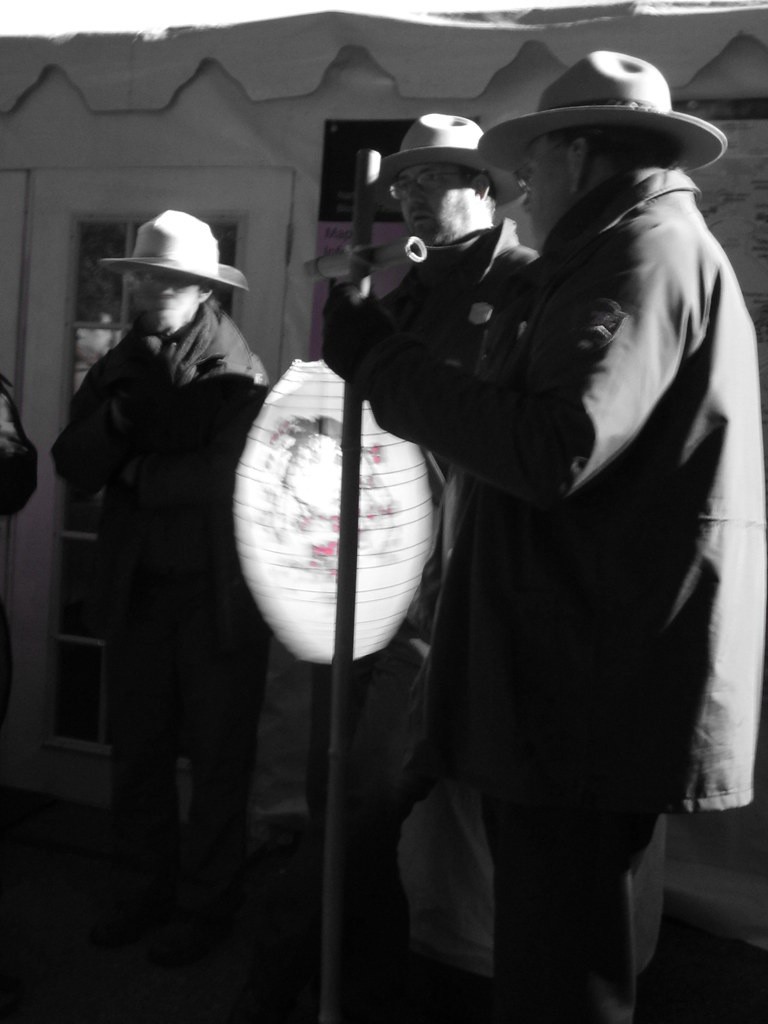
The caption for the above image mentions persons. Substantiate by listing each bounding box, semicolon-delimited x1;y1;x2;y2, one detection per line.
312;45;768;1024
0;371;37;724
42;208;270;972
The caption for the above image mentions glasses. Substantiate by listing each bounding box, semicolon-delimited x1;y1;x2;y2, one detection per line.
513;131;584;194
122;264;193;290
388;171;468;201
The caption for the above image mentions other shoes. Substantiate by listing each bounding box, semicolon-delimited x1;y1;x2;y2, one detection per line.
229;963;314;1024
89;886;176;949
148;909;232;967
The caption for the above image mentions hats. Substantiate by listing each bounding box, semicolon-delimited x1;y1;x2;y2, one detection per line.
365;113;527;216
97;209;250;292
476;49;729;173
